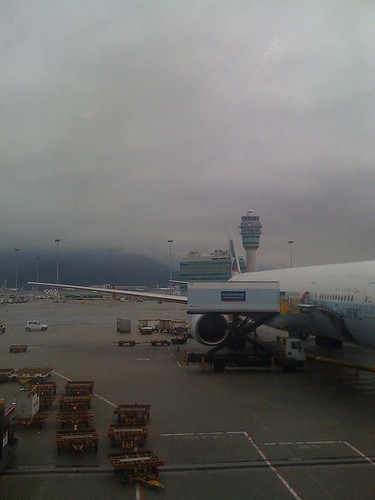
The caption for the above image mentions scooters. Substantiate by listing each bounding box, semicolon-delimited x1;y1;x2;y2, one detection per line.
1;324;6;333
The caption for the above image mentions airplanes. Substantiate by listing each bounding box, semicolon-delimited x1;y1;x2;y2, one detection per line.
26;260;375;373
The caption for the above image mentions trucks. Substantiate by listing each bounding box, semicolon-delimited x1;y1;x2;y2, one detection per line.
117;317;131;334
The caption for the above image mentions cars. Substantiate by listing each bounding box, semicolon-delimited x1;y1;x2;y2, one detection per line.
141;325;153;335
26;320;48;331
